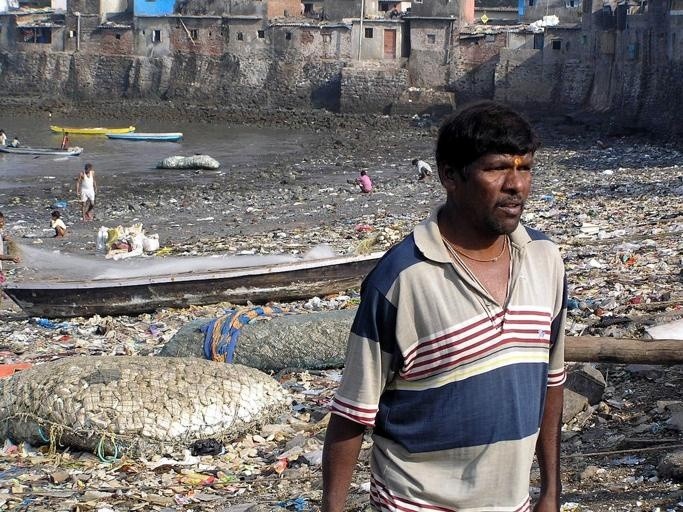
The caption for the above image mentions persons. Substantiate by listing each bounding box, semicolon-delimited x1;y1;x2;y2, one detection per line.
412;158;433;179
75;164;97;222
0;212;20;288
321;101;566;512
357;171;372;193
384;4;398;19
49;210;66;238
61;132;69;150
0;129;20;148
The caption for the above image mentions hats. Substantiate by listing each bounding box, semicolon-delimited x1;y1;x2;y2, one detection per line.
51;211;62;217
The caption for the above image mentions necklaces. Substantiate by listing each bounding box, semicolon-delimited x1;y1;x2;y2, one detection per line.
440;231;507;262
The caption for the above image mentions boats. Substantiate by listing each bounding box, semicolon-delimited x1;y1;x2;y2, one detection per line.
1;144;83;158
50;124;135;135
106;132;185;143
